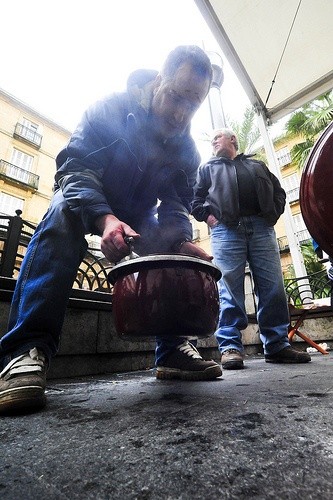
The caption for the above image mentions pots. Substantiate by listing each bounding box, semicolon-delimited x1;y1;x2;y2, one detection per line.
107;238;222;340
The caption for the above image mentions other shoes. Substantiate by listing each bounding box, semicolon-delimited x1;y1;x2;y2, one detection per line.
155;342;222;381
1;348;48;410
264;347;311;363
221;348;243;369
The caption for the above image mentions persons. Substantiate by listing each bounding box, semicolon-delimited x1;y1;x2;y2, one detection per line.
0;44;224;412
311;238;333;315
190;127;312;370
